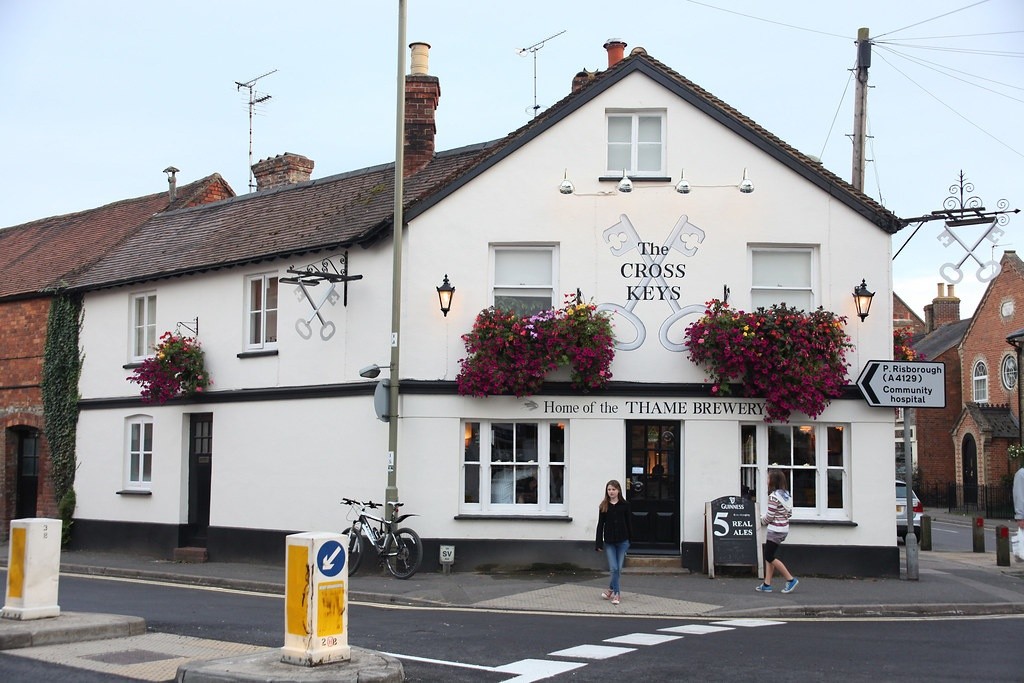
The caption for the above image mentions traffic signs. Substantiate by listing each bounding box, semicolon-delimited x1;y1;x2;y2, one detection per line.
856;360;946;408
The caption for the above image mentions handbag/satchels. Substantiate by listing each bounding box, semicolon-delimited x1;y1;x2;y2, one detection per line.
1011;525;1024;560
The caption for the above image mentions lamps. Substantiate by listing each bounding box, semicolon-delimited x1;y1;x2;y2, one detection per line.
618;169;633;192
560;169;575;194
740;168;754;192
436;274;455;317
675;169;691;193
852;279;875;322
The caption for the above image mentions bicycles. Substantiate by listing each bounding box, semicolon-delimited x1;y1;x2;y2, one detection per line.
340;498;422;579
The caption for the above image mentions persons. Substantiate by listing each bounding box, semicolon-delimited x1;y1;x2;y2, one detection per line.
491;459;563;505
1013;466;1024;562
595;480;634;603
756;470;799;594
645;464;668;499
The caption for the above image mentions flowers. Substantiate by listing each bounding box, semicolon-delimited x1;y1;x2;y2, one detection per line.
126;331;214;406
893;326;928;362
455;294;618;399
684;299;857;424
1008;443;1024;462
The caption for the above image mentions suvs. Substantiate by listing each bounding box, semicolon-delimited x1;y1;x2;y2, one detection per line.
895;477;923;543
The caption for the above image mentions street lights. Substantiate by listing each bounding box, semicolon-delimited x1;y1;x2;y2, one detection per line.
359;363;399;574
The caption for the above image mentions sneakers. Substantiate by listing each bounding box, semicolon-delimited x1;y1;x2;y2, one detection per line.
756;582;773;592
601;589;612;598
781;578;798;594
611;592;620;604
